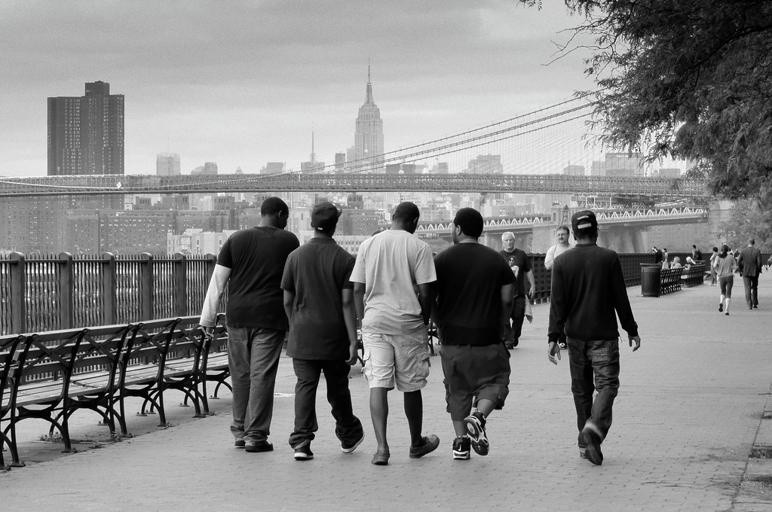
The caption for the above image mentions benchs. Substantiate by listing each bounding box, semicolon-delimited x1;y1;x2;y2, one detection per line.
355;317;438;372
0;309;236;477
658;263;706;296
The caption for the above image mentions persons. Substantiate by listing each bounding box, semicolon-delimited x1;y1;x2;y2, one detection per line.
426;207;518;458
547;211;641;464
652;237;763;315
199;197;300;454
347;202;437;462
496;230;536;349
280;201;365;459
544;225;574;349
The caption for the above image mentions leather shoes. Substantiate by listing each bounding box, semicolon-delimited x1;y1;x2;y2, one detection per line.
372;453;390;465
409;435;440;459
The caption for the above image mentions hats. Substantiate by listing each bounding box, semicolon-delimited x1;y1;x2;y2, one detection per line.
573;210;597;231
310;201;342;231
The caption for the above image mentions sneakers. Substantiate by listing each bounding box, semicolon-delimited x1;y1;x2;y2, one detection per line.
582;428;603;465
342;434;364;453
463;408;489;456
245;439;273;452
235;439;245;446
578;432;588;461
294;444;313;459
719;303;724;312
452;437;471;460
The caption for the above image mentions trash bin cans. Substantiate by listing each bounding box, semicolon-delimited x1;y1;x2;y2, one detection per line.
640;263;662;297
